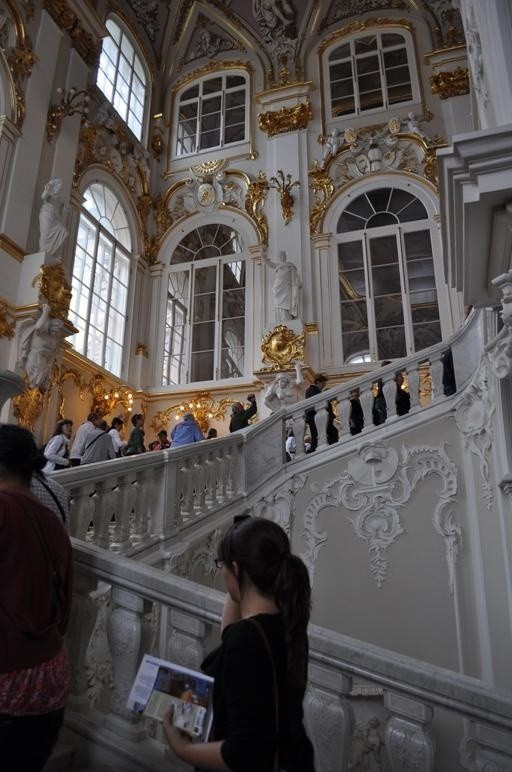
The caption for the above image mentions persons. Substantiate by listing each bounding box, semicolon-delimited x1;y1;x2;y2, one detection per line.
23;304;63;390
0;424;73;772
441;349;456;396
229;394;258;432
263;362;305;411
321;127;344;162
259;248;302;322
28;449;71;538
398;112;437;145
162;514;315;772
41;412;217;535
285;360;411;461
38;178;69;258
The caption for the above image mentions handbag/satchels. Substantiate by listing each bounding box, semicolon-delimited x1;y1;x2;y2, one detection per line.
37;444;48;471
123;444;139;455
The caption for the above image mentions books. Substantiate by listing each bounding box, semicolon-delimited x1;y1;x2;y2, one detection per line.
124;652;217;742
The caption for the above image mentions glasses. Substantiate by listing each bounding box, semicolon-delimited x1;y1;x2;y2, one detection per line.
213;559;224;569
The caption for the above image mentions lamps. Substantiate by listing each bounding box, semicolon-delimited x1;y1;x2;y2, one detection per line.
345;349;373;363
46;87;94;144
174;399;214;433
92;389;134;424
264;168;301;227
